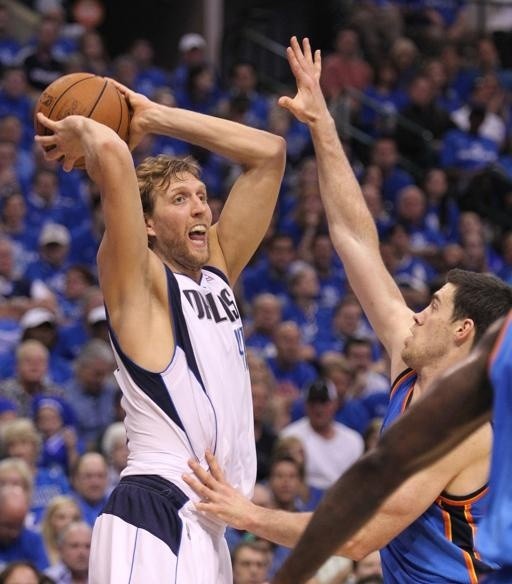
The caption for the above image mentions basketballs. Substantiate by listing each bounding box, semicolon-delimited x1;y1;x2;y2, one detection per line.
33;73;130;170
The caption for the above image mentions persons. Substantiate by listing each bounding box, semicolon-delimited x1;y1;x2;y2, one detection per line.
1;1;509;581
273;316;512;583
183;37;509;583
35;76;287;583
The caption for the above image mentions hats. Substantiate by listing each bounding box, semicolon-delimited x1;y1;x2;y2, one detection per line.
37;222;72;249
306;377;339;404
19;306;55;339
87;304;107;326
178;32;208;53
